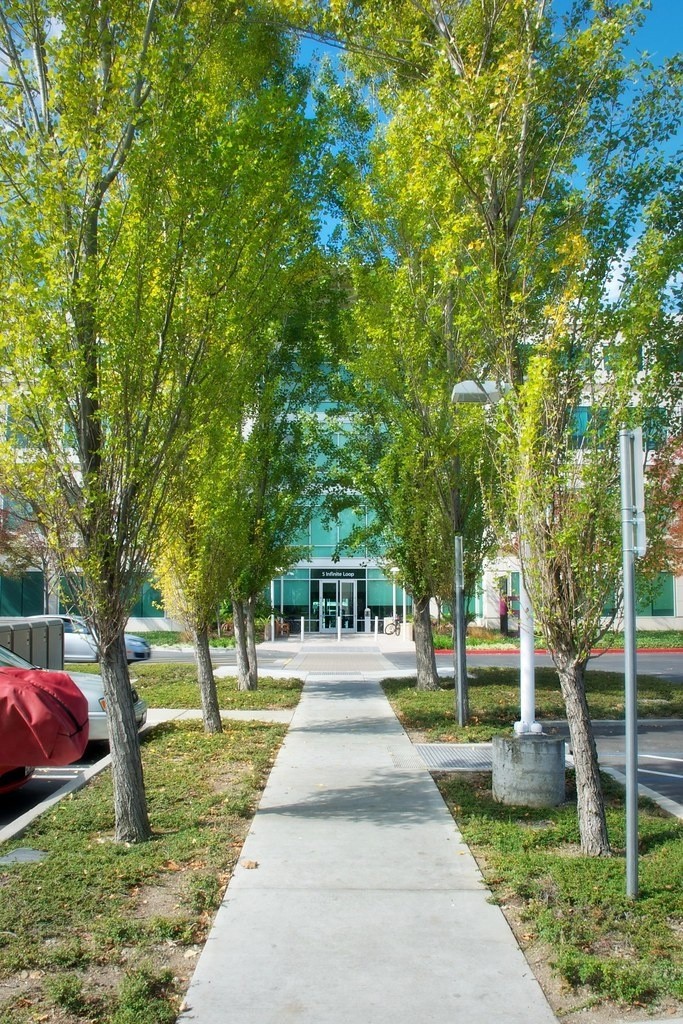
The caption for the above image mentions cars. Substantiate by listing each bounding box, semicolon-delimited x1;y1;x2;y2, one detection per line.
28;614;151;662
40;665;147;740
0;642;90;803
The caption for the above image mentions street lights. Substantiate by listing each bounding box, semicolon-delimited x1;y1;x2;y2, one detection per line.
452;375;545;734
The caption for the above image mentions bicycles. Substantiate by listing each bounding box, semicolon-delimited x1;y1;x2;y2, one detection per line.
385;620;400;636
